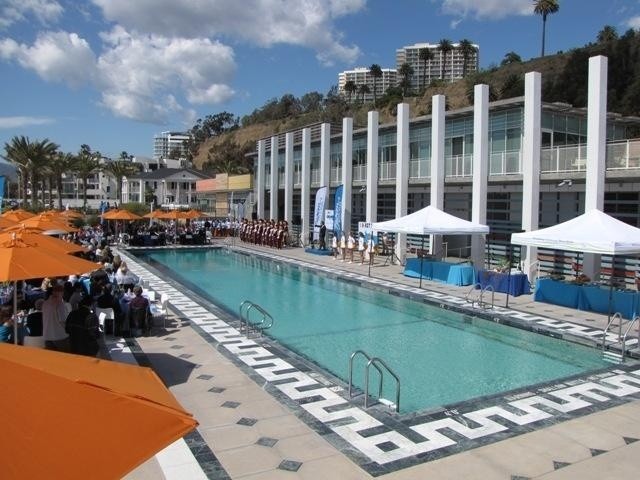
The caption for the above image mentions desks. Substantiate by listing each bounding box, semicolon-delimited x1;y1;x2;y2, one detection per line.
402;257;639;321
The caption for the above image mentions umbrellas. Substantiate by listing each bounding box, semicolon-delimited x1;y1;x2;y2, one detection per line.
0;208;208;242
0;342;200;480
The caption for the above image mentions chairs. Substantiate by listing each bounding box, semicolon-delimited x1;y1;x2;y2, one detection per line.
22;270;171;350
126;228;212;245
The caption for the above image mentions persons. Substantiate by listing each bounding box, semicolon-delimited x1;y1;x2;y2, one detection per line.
203;218;288;251
1;219;201;358
315;221;326;250
332;231;375;267
382;232;389;253
